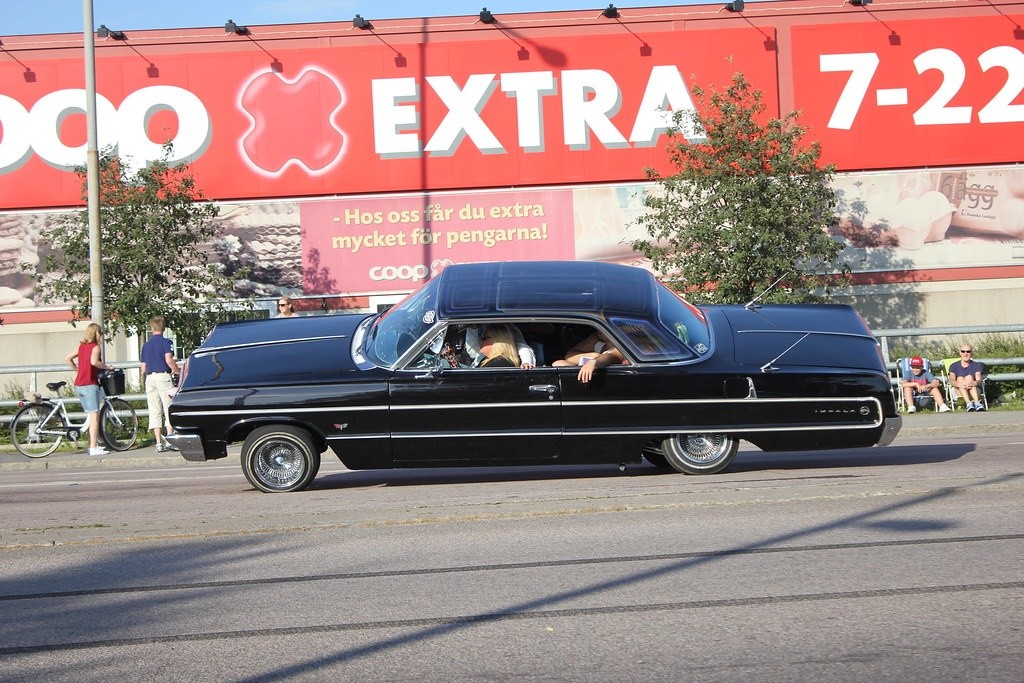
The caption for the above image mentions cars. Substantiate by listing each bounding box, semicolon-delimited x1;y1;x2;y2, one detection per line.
166;260;903;494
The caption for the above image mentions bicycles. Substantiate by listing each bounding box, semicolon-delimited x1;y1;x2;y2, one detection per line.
9;369;139;458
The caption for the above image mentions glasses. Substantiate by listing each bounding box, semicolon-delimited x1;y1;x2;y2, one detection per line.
480;334;490;341
960;350;972;354
279;303;289;306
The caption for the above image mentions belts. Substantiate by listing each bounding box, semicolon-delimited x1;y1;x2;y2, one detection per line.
148;371;172;375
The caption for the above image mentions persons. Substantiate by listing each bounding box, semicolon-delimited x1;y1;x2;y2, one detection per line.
902;356;951;414
948;345;985;412
66;323;115;456
140;316;181;453
441;324;630;383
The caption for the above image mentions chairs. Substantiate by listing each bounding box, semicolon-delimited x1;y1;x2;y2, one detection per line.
939;358;989;412
895;358;942;413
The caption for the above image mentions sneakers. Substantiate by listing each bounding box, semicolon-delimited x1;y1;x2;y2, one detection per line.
907;406;916;414
966;401;975;412
165;442;180;451
974;402;986;411
939;404;951;412
155;443;164;451
87;442;110;455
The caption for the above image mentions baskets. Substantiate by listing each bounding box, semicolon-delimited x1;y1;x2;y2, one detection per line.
100;373;125;397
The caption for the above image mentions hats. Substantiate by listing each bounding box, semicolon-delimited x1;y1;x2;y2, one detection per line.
910;356;924;367
